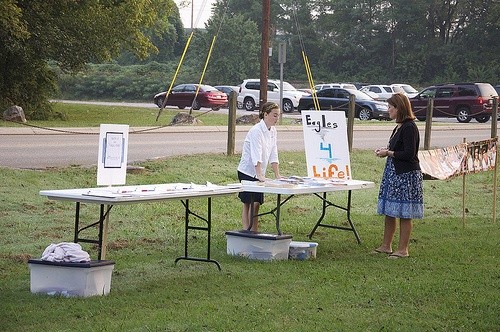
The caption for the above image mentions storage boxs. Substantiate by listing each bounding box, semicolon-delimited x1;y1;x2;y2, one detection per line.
289;241;318;260
226;230;294;260
28;260;116;299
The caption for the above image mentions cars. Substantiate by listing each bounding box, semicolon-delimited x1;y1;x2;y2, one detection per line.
154;83;229;112
213;85;244;109
298;87;392;122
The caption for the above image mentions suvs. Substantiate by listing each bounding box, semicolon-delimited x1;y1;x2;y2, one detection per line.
409;82;500;123
296;82;357;95
359;83;419;99
236;79;312;113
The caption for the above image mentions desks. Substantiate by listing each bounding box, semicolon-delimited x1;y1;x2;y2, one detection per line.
229;177;375;245
40;183;243;271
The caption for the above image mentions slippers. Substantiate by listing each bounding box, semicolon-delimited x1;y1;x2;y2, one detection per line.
372;248;392;253
389;252;408;257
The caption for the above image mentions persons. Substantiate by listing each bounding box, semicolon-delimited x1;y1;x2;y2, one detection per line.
370;93;423;261
237;101;287;232
